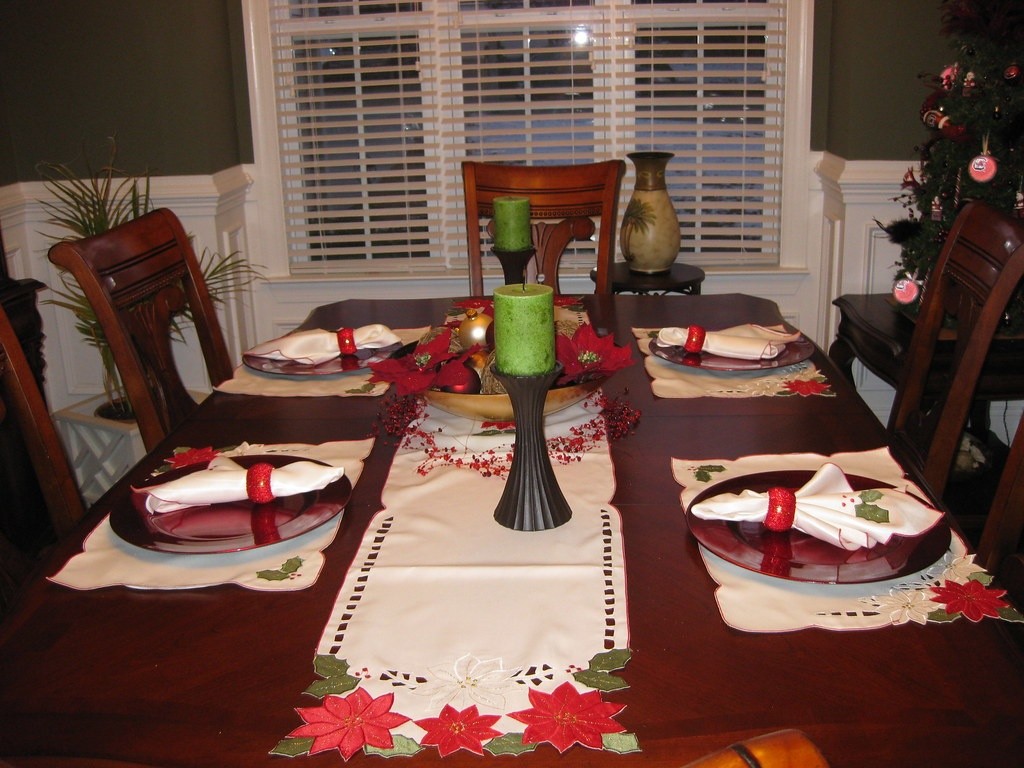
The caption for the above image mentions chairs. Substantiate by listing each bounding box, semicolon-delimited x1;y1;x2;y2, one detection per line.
47;208;234;455
656;323;800;361
976;411;1024;639
462;159;626;296
0;308;86;581
884;200;1023;488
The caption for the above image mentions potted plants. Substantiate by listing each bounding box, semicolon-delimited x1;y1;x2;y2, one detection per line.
30;130;269;507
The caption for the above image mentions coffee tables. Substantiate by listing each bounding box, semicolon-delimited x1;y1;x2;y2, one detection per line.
590;263;706;295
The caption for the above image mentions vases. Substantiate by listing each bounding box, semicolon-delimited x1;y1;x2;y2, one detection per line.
620;152;682;272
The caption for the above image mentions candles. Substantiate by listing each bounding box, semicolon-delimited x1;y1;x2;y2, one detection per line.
495;284;554;376
493;197;531;252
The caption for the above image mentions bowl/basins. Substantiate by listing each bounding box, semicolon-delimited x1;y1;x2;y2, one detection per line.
418;340;622;423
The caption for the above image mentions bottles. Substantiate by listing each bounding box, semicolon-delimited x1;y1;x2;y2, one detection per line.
620;152;681;277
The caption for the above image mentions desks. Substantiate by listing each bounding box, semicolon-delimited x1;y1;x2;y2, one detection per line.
829;293;1024;501
0;274;1024;768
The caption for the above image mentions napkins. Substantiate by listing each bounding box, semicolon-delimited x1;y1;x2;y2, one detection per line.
693;463;947;551
129;456;344;514
241;324;401;366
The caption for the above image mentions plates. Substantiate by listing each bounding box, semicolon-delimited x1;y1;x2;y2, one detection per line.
109;455;353;555
243;324;402;375
686;470;951;583
650;336;814;371
